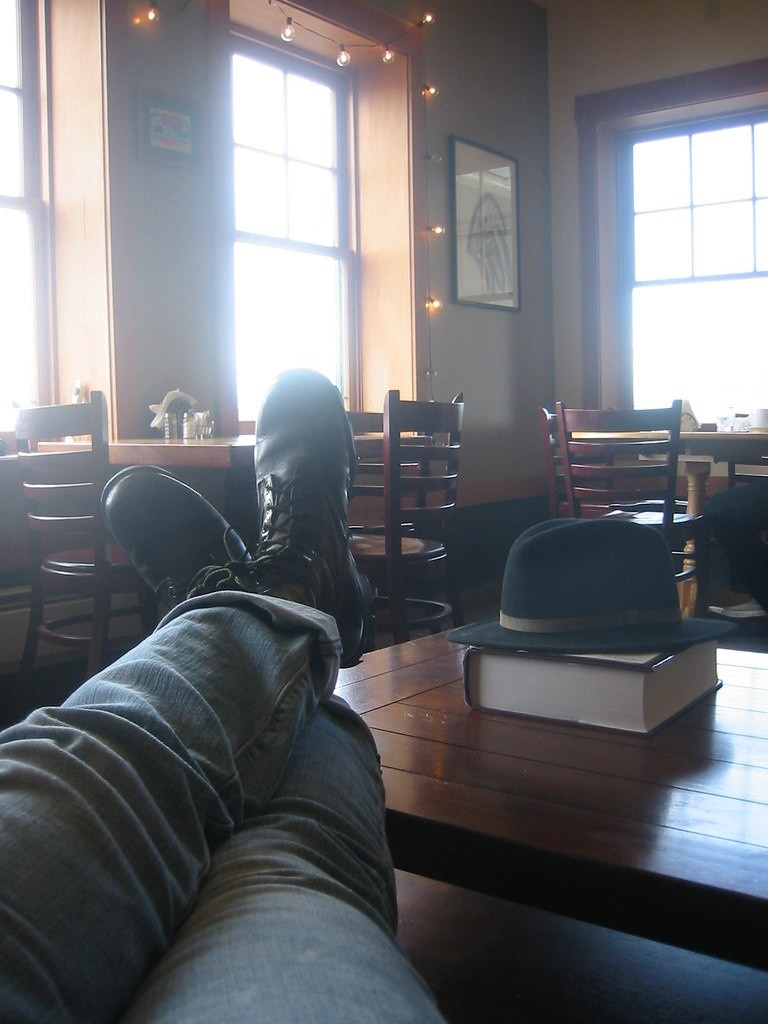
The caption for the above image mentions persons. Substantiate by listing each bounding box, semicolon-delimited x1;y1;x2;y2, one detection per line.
0;367;441;1024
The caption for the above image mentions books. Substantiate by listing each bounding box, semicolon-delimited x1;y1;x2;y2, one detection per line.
462;637;723;736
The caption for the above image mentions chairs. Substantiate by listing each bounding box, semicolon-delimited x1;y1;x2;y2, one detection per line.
11;390;161;684
346;388;466;640
540;401;705;606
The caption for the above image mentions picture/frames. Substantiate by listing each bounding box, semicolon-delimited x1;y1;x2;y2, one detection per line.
448;136;523;313
135;83;207;167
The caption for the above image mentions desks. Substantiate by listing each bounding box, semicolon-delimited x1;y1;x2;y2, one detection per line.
36;436;445;552
572;430;767;619
335;619;768;971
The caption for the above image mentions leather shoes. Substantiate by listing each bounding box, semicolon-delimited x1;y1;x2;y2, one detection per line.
100;462;253;604
200;367;375;670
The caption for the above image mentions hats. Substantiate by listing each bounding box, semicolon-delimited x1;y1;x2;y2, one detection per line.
445;516;737;653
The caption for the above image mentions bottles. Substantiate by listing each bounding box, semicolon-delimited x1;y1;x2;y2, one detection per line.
163;411;178;439
182;412;196;438
